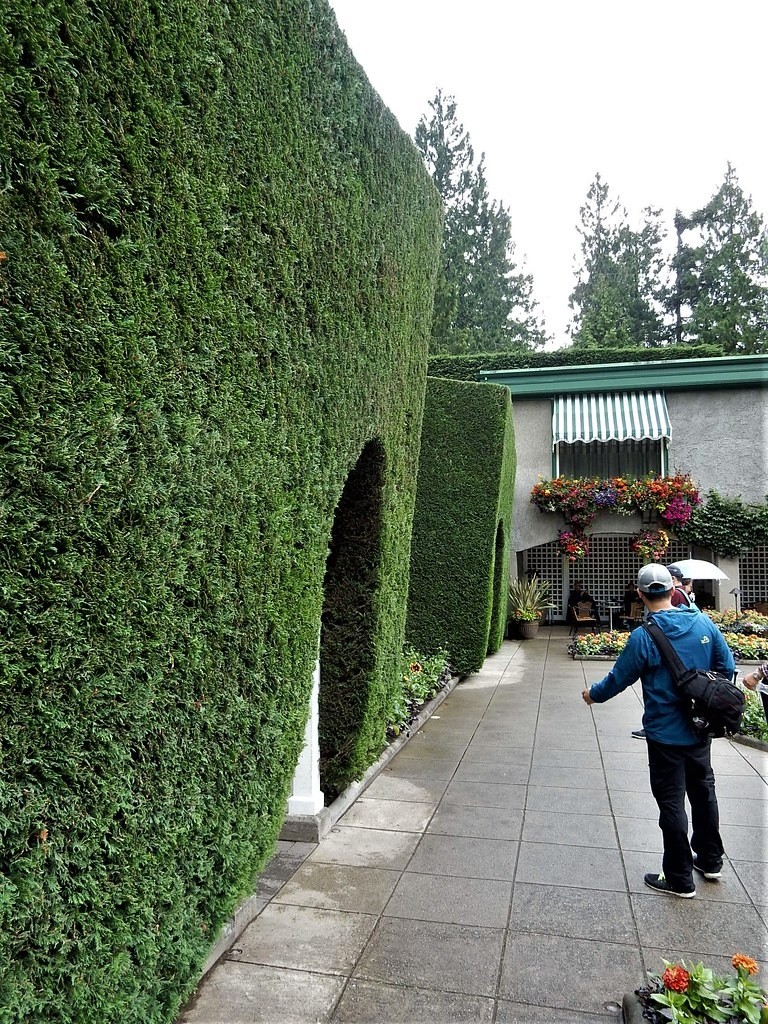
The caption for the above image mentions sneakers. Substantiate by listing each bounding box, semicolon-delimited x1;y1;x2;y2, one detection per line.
644;873;697;898
693;853;724;879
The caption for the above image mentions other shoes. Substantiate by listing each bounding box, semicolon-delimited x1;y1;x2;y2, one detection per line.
632;728;647;739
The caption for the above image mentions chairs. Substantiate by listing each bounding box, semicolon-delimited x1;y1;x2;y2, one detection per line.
619;601;646;631
568;601;600;638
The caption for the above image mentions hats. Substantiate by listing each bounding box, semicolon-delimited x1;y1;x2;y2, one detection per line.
666;565;684;577
636;563;673;592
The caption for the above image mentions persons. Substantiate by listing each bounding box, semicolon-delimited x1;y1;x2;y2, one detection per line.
581;562;736;898
565;581;604;630
742;661;768;725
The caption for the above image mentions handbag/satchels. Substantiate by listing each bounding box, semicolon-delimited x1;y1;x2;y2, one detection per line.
674;667;746;738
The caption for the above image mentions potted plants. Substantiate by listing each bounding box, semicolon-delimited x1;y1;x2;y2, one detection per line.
508;572;557;640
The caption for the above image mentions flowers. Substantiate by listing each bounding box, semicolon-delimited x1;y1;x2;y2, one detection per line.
530;470;705;560
509;609;542;622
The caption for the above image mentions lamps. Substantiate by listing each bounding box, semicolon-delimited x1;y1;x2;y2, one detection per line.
729;588;743;613
733;667;743;684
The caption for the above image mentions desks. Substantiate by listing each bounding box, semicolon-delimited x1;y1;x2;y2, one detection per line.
601;605;624;631
604;601;617;606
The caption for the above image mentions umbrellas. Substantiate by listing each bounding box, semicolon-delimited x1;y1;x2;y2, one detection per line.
666;551;729;603
